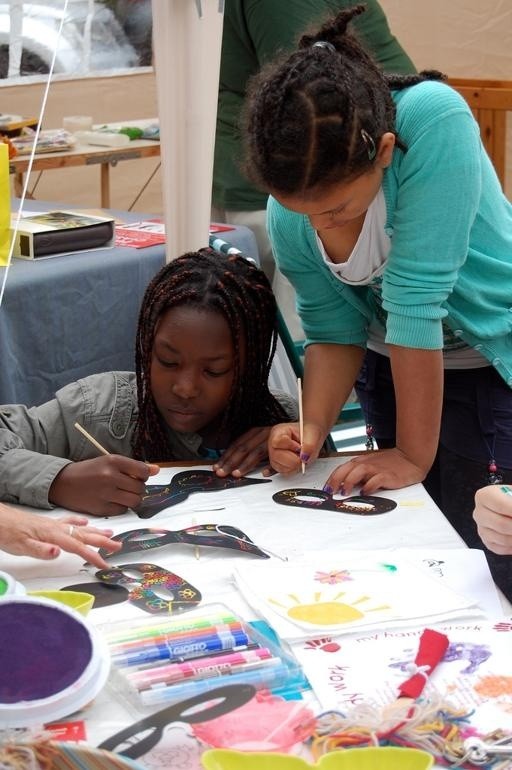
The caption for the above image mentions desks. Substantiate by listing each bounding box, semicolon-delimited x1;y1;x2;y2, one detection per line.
0;454;511;769
0;192;261;407
1;118;161;209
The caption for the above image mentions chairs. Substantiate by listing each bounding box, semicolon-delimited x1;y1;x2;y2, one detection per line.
210;233;382;453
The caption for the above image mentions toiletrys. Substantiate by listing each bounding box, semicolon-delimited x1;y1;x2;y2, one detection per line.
74;129;130;147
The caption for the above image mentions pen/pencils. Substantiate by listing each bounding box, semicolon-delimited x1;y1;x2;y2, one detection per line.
74;422;149;494
297;378;306;476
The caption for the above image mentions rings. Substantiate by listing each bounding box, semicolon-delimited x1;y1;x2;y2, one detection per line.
69;523;74;537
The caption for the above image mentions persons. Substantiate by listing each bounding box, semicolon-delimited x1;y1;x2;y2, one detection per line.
470;484;511;603
0;501;124;572
0;245;293;519
211;0;420;423
239;2;512;530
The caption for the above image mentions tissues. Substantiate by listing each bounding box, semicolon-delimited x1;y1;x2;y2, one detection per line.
0;113;41;139
